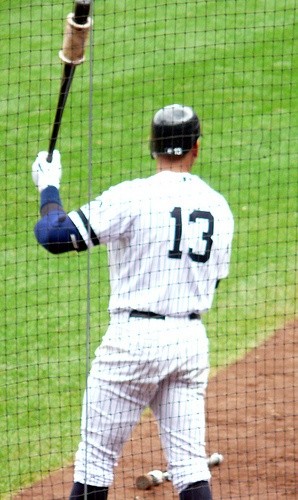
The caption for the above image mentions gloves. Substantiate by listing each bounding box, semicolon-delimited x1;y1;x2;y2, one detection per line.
30;150;63;207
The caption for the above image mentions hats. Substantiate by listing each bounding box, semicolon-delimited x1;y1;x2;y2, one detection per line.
149;103;202;160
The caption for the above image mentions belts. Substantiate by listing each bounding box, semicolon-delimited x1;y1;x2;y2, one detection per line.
115;310;204;322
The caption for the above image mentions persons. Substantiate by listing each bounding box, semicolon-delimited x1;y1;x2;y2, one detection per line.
31;104;235;500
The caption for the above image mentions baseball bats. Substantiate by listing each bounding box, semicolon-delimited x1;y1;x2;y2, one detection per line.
46;0;93;161
136;452;226;490
